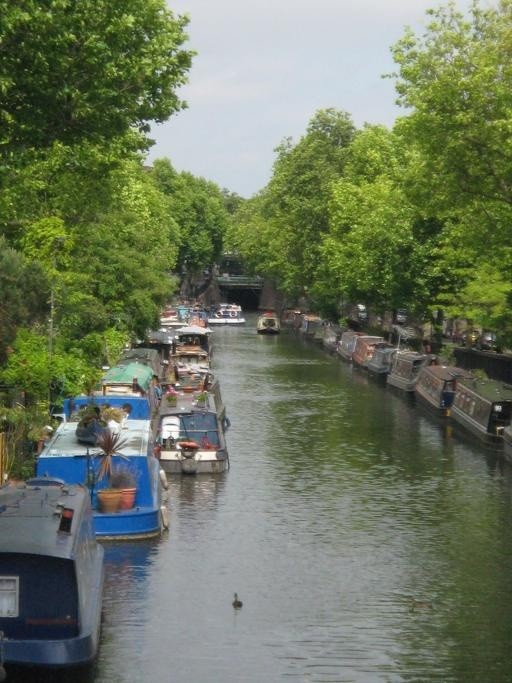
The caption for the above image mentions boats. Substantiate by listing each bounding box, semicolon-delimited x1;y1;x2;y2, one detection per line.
255;310;282;333
114;348;163;381
35;395;168;544
163;371;226;424
138;299;214;371
152;404;231;476
207;301;246;324
283;304;512;461
100;363;165;402
0;477;108;682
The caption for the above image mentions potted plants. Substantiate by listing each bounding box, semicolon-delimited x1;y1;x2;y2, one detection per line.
96;472;138;512
